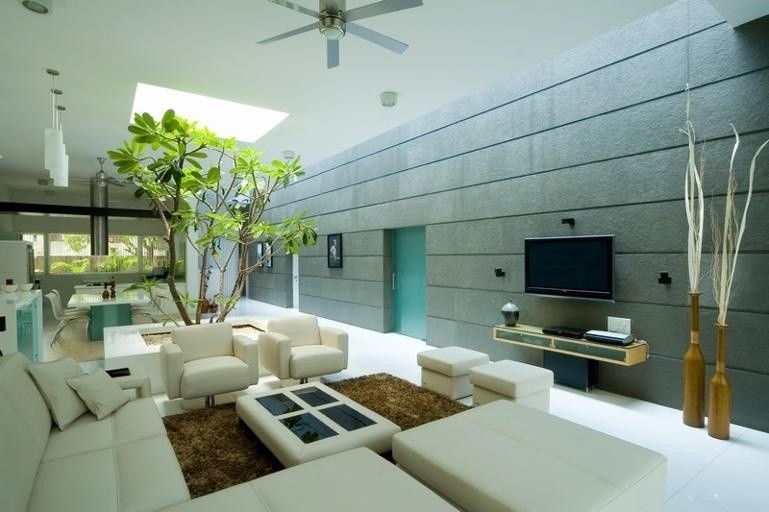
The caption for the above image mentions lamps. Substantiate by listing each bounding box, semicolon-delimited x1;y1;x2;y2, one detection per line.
319;0;345;40
43;68;71;187
95;181;106;186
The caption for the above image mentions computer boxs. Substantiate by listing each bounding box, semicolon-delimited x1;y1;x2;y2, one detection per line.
542;349;600;392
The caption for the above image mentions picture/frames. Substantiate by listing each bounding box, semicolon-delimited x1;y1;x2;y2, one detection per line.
327;233;342;268
256;240;272;268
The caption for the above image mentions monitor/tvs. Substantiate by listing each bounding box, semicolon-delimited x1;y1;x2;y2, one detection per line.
523;234;616;304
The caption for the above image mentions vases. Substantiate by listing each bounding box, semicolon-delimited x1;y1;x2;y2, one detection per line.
708;321;731;441
683;291;705;429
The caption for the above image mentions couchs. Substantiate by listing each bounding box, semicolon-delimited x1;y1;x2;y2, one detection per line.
0;351;458;511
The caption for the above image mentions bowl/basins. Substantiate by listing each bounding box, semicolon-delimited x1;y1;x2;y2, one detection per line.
2;285;16;294
20;284;34;292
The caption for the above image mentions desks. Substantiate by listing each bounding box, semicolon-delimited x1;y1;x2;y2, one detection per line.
0;289;43;359
67;292;151;341
494;321;647;393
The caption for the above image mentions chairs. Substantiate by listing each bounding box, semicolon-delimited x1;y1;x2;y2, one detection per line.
44;288;90;347
129;288;163;323
258;315;348;385
160;322;258;409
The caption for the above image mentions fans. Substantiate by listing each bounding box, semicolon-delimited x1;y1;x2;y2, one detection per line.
256;0;423;69
69;157;135;187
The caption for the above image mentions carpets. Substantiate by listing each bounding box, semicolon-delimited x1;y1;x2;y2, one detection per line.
162;373;473;499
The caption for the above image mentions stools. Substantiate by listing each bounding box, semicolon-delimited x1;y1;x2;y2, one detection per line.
418;346;488;400
392;396;666;512
469;359;554;413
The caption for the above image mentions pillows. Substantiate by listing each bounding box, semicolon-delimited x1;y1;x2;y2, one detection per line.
27;355;131;430
